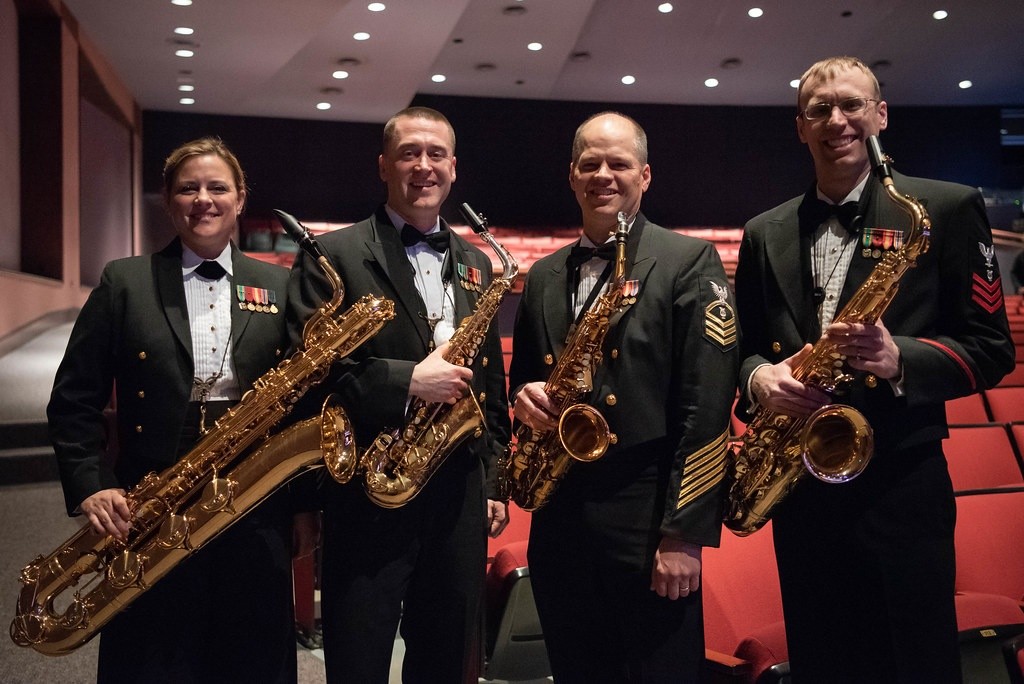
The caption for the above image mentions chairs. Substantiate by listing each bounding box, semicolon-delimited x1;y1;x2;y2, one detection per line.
236;218;1024;684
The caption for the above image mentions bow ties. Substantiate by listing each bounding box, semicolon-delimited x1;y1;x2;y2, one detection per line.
571;243;617;268
400;223;449;253
814;201;859;230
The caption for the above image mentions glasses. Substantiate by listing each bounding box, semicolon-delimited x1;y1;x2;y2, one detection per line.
798;98;880;121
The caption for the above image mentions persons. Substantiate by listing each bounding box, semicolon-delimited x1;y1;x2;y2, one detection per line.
284;107;512;684
735;55;1015;684
508;110;740;684
46;137;298;684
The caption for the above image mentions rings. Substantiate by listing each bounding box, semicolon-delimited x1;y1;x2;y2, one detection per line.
856;346;862;360
680;588;690;591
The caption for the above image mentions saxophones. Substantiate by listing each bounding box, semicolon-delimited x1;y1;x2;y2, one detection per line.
713;131;933;537
354;200;521;513
497;209;647;514
6;205;402;656
